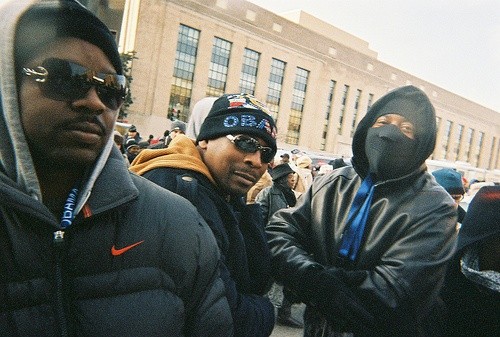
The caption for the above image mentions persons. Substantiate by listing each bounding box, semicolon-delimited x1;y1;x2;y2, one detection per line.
0;0;234;337
128;89;276;337
266;86;457;337
245;147;350;325
113;118;188;170
449;185;500;337
429;167;500;234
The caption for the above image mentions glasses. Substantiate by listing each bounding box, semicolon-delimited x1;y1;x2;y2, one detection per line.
18;56;130;112
451;195;463;201
225;133;276;164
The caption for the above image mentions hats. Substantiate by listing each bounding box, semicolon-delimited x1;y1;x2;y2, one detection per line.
271;162;296;181
192;92;279;156
317;164;334;176
431;167;465;196
280;153;289;159
170;119;186;135
126;137;140;150
128;124;136;132
296;155;312;168
